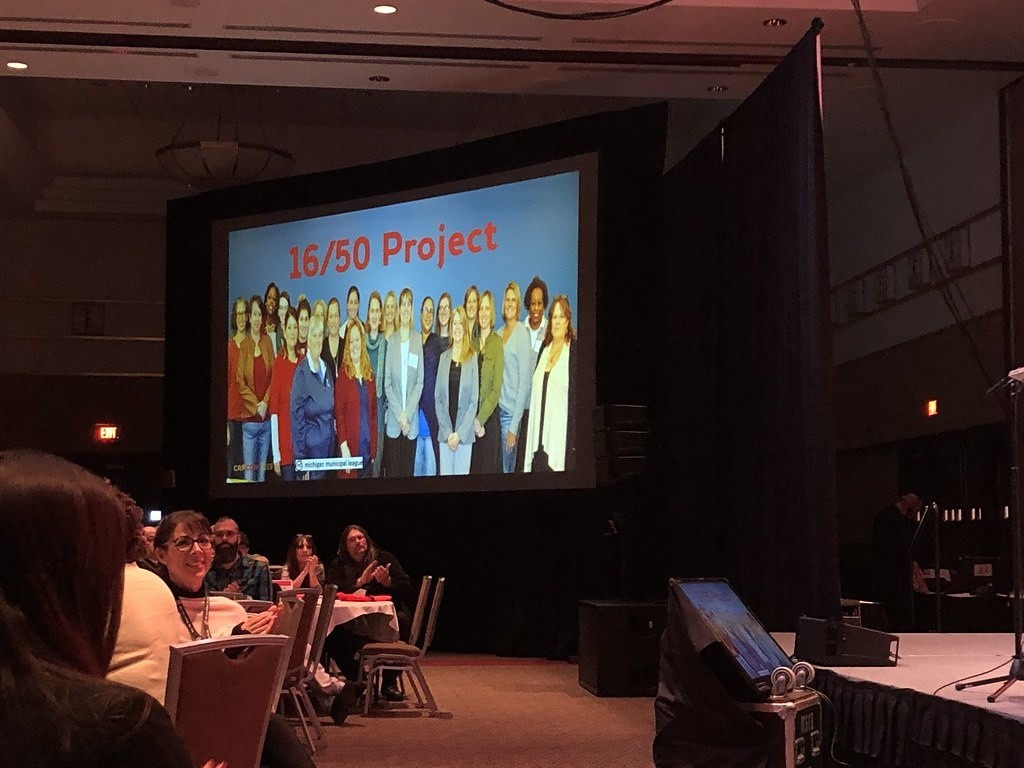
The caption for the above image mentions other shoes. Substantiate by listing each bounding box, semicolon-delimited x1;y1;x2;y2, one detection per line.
379;684;404;700
329;678;368;726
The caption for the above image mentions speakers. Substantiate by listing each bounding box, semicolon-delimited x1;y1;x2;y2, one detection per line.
577;599;668;697
793;615;899;668
592;403;652;488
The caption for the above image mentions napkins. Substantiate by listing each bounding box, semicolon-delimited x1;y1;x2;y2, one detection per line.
339;593;372;602
370;594;393;601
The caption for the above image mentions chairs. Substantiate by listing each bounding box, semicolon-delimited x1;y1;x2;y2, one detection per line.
164;634;293;768
359;577;446;718
357;575;432;709
279;582;339;738
209;588;324;756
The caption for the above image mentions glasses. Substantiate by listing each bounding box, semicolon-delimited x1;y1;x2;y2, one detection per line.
160;534;216;552
422;306;434;315
296;534;313;539
236;311;245;319
438;305;450;312
553;294;568;300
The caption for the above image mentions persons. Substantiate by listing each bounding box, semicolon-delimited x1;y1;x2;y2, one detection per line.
226;275;578;483
153;510;316;768
869;485;928;630
1;448;232;768
135;508;412;724
90;474;190;712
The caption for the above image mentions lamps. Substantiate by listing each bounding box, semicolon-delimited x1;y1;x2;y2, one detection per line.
155;81;295;189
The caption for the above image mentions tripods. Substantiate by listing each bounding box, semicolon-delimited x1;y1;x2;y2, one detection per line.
955;367;1024;704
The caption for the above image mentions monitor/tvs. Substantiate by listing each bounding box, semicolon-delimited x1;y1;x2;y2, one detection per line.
670;578;796;702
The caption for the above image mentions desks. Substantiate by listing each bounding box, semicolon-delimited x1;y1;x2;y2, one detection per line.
313;593;399;676
